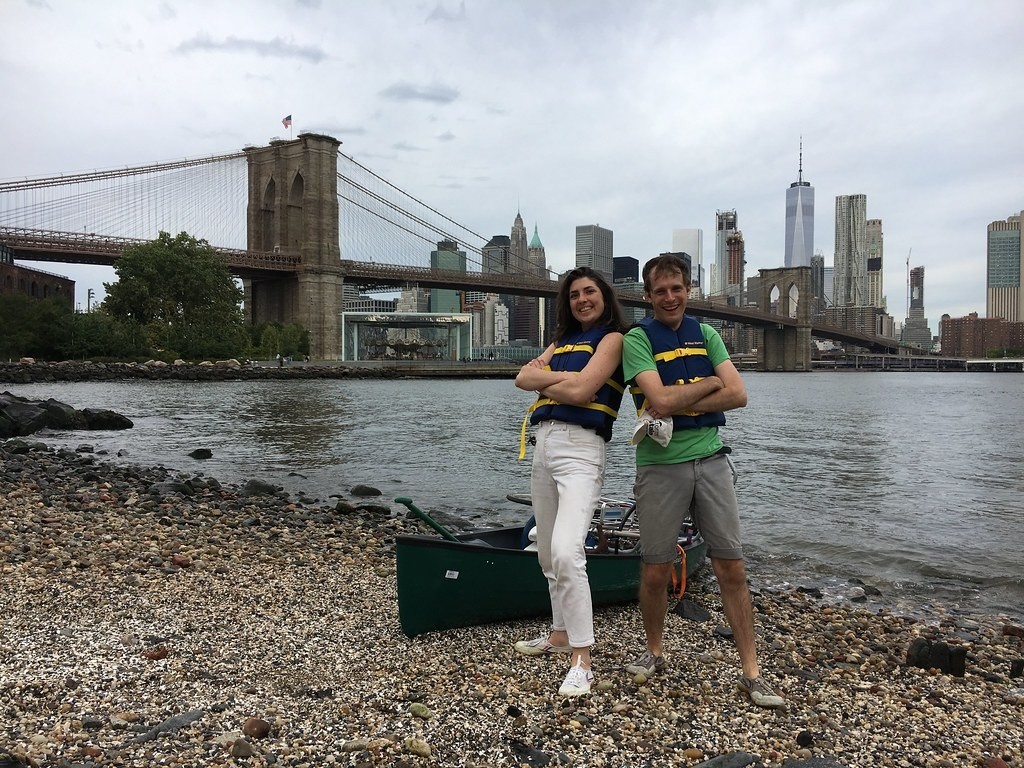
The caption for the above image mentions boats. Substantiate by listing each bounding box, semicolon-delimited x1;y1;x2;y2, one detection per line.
392;450;738;638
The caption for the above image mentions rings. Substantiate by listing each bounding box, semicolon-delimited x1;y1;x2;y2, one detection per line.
653;411;657;415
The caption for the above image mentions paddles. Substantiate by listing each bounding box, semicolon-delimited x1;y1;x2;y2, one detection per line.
394;497;461;542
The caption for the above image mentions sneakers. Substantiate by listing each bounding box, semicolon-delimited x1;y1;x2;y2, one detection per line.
514;636;573;655
627;650;669;677
737;673;784;706
558;665;594;696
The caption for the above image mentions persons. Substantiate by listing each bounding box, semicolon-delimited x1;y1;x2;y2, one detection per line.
621;253;787;709
513;265;625;699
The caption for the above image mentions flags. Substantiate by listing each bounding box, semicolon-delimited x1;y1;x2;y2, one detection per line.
282;114;292;129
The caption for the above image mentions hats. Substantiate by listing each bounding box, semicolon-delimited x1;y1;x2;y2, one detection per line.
632;408;674;448
